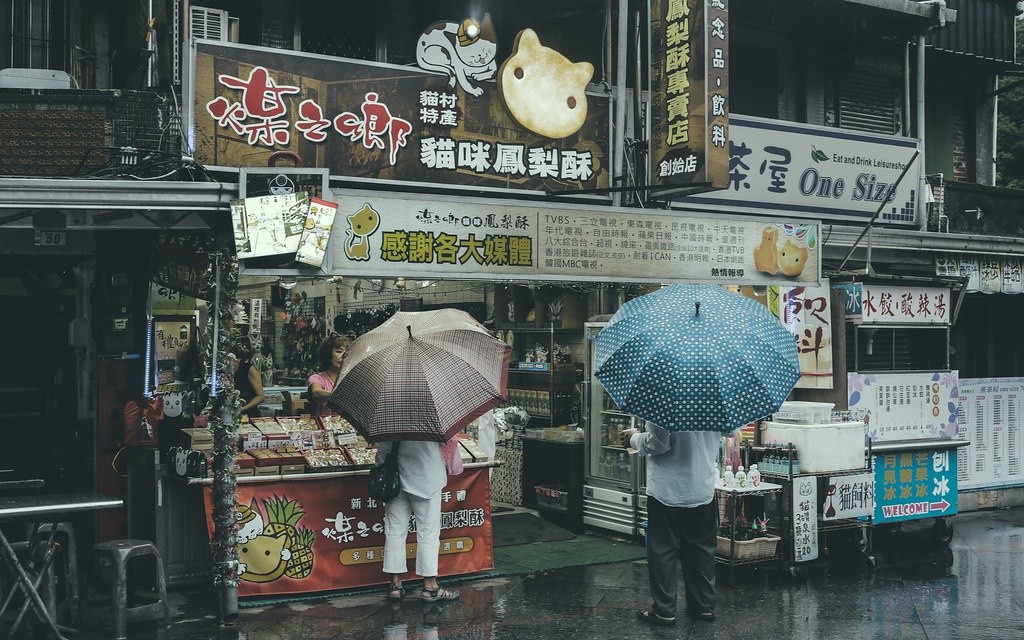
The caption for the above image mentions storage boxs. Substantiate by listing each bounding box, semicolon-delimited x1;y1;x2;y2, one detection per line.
760;401;866;473
493;281;588;331
182;400;488;476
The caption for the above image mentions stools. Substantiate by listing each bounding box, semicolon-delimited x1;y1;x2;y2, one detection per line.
0;520;172;640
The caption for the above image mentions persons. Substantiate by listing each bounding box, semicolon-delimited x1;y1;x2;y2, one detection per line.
619;422;739;626
229;355;265;418
375;437;461;601
309;337;353;418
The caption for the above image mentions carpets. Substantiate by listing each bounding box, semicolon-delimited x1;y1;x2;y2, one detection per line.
491;511;576;548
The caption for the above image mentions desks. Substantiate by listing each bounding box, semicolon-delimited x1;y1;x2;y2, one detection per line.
0;491;124;640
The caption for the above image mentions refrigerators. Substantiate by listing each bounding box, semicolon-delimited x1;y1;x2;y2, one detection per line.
583;322;636;535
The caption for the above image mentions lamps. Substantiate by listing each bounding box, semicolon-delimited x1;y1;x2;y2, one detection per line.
278;275;297;289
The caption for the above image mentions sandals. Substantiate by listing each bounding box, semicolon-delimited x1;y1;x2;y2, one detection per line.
636;608;676;625
388;585;406;599
686;606;716;621
420;583;460;602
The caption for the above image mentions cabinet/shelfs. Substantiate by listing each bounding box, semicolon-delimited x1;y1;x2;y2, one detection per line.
490;323;583;429
714;437;873;584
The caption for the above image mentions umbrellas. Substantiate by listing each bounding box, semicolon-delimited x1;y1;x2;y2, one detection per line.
326;305;513;443
595;283;804;434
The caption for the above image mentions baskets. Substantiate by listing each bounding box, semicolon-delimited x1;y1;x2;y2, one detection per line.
714;530;781;560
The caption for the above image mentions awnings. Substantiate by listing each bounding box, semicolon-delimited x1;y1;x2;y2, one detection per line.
0;175;241;230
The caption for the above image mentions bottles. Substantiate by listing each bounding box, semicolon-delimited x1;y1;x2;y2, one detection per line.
601;420;609;447
723;466;734;489
762;442;799;466
715;463;720;488
618;419;625;447
748;466;758;489
735;466;746;490
614;419;619;445
610;417;614;446
752;464;760;488
626;419;631;429
603;392;617;411
599;452;632;482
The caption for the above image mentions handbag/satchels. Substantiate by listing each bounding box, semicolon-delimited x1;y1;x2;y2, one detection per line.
442;435;464;475
367;453;400;502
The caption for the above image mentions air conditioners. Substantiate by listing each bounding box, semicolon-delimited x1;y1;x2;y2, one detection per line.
189;5;240;43
